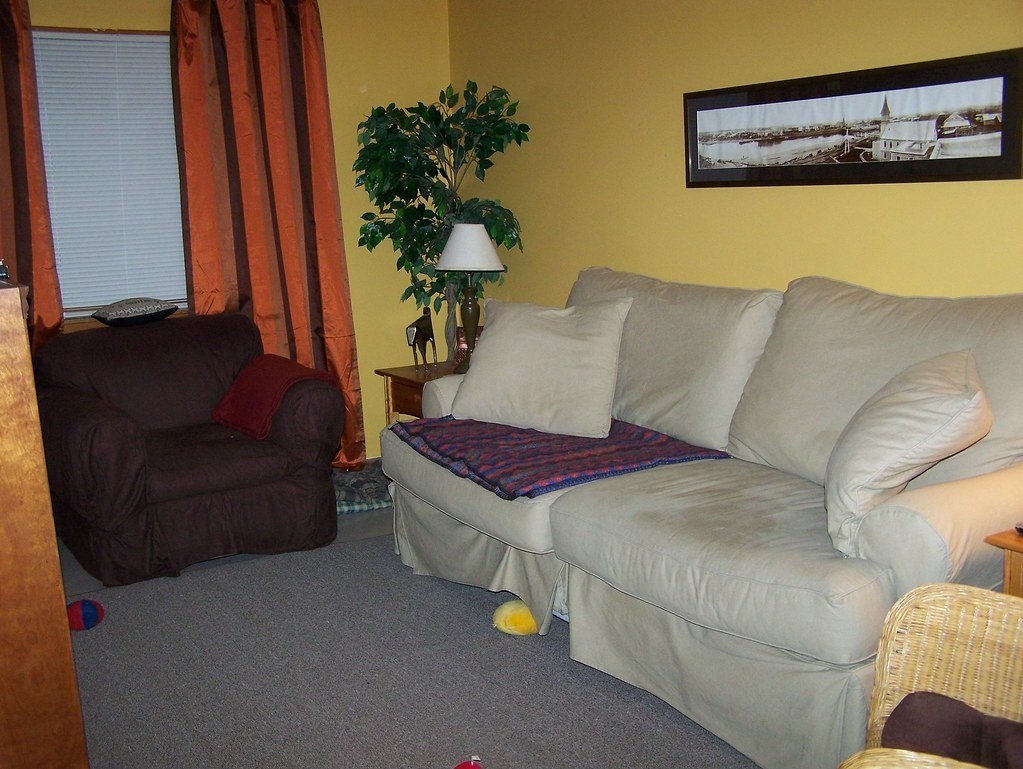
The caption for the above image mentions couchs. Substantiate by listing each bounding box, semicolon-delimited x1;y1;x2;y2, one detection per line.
34;314;346;589
380;267;1023;769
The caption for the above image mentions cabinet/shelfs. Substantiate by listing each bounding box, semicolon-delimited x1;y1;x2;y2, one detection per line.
0;280;89;768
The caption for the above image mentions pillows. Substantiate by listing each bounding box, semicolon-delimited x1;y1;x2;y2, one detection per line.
452;296;634;439
823;347;994;558
92;297;179;327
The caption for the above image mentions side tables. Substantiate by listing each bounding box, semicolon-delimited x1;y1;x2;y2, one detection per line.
984;528;1023;598
375;362;460;420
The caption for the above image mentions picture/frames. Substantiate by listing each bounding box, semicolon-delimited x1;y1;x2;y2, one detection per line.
684;46;1023;188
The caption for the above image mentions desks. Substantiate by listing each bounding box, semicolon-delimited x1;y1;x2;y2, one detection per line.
836;748;988;769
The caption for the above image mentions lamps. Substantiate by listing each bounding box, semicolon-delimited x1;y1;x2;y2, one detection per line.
435;223;504;373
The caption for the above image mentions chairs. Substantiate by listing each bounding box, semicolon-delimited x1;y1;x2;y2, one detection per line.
835;582;1023;769
